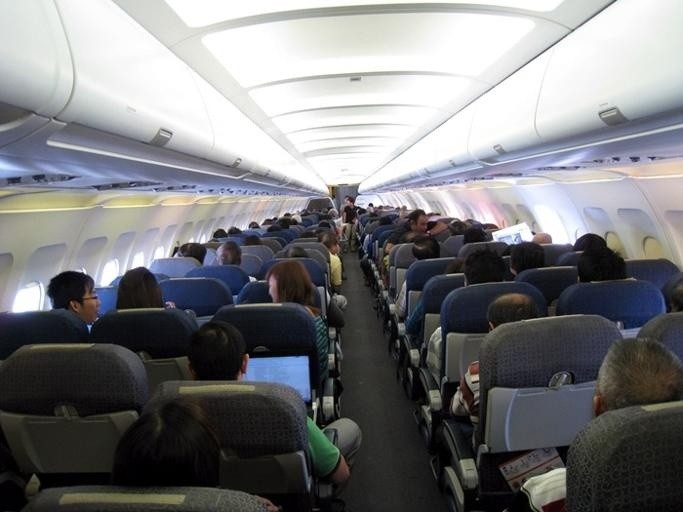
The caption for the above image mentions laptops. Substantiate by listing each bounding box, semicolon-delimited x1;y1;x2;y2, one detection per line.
241;355;310;413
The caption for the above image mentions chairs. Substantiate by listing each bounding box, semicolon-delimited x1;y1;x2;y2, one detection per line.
2;205;347;512
352;206;682;511
